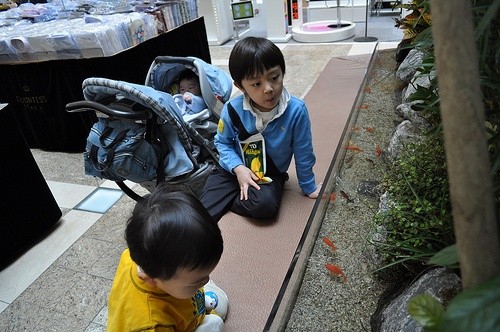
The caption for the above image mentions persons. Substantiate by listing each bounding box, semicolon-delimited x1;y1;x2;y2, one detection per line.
104;186;229;332
195;36;326;223
171;69;206;123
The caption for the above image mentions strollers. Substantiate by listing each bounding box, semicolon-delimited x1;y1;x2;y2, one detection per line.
65;56;234;204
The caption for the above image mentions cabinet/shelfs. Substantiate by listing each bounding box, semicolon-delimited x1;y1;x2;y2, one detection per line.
0;15;212;154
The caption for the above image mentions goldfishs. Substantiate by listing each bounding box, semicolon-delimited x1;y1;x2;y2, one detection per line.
352;126;376;135
345;144;364;153
365;59;383;94
323;263;348;284
330;189;355;205
373;145;383;157
322;238;336;253
355;104;371;109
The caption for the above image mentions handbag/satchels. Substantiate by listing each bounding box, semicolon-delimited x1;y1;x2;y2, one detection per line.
83;119;165;202
